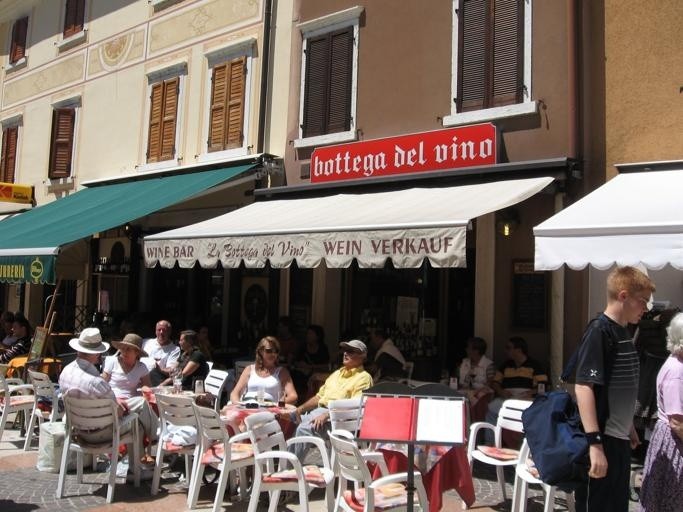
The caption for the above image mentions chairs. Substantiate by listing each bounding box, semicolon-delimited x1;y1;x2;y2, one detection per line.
0;371;36;442
324;429;430;511
234;359;255;385
203;368;229;417
205;362;213;371
243;409;336;512
187;400;277;512
54;395;142;505
235;466;251;501
326;398;392;487
150;392;201;508
23;368;64;453
510;436;577;512
459;397;537;512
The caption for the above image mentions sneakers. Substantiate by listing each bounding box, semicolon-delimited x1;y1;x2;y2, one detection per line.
128;466;155;482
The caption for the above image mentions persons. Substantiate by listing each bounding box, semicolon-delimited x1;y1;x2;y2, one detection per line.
486;336;549;446
0;312;16;344
561;265;657;512
102;333;153;397
371;329;406;378
0;317;31;363
301;324;330;374
451;336;496;389
3;317;18;346
144;319;180;384
153;329;206;390
231;336;298;404
59;328;160;483
639;312;683;512
289;339;374;468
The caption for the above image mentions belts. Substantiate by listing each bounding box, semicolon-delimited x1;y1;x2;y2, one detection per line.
79;428;109;434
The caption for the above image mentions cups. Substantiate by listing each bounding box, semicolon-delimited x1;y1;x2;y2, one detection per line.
257;385;264;404
174;387;185;395
278;391;285;408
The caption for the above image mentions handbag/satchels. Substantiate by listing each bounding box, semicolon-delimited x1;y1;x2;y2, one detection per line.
522;316;616;489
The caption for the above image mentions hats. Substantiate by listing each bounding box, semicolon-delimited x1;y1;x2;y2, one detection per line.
67;328;112;356
341;339;366;354
114;334;149;358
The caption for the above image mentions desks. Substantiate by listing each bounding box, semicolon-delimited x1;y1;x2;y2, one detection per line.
365;438;478;512
221;402;301;501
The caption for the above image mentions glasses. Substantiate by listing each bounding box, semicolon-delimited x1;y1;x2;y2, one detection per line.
263;347;281;354
